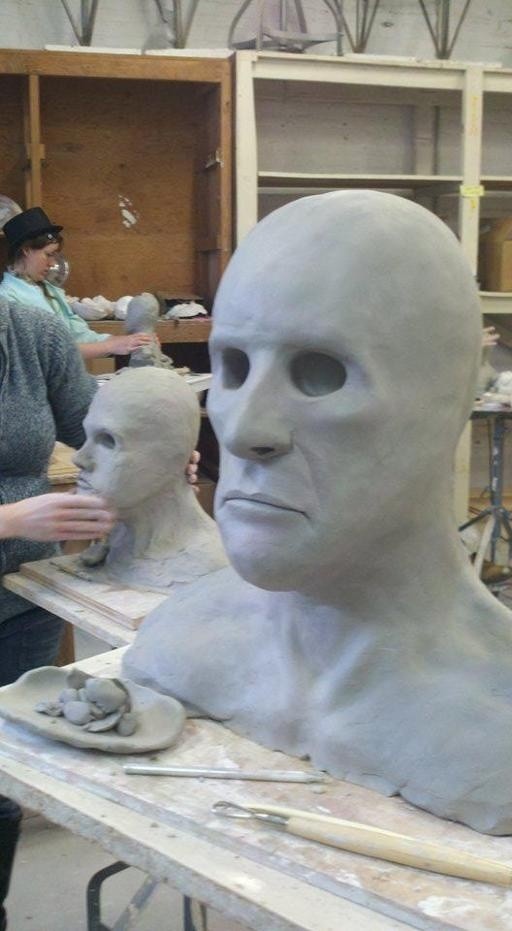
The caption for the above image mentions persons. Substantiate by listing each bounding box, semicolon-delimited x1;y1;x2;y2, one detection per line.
116;184;512;839
122;290;175;367
2;293;206;931
0;205;162;361
64;359;225;595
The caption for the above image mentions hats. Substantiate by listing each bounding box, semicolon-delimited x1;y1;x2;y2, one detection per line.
2;205;65;251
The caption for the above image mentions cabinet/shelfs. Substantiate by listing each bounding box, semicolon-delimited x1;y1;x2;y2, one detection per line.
0;43;234;518
233;49;512;589
